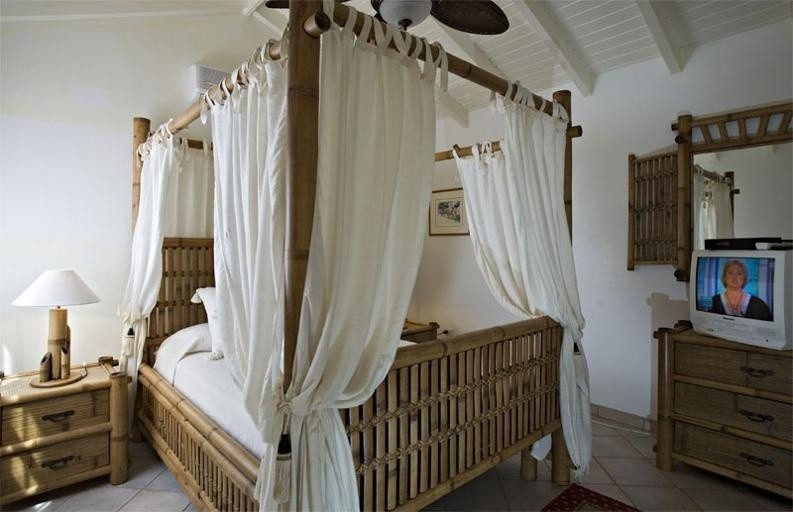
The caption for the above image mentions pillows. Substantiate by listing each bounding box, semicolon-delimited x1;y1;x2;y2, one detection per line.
190;287;224;360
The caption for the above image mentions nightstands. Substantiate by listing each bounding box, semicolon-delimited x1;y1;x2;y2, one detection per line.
0;356;132;505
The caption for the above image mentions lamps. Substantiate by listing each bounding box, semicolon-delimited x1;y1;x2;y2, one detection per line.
10;268;100;388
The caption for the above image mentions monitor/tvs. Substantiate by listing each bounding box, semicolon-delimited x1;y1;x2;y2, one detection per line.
689;250;793;351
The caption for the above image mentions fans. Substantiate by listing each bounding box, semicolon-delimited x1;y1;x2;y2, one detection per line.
265;0;510;35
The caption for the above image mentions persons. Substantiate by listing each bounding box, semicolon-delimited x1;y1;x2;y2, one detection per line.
712;259;771;319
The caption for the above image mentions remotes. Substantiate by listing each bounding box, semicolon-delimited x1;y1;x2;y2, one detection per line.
755;242;793;250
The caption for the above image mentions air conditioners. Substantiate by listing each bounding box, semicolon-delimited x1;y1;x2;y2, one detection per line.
188;64;233;103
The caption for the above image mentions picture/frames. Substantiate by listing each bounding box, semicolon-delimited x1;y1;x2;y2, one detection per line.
429;188;470;236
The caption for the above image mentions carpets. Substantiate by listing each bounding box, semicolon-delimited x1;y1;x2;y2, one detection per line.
539;483;644;512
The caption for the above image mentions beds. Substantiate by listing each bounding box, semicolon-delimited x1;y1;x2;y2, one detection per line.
127;0;582;512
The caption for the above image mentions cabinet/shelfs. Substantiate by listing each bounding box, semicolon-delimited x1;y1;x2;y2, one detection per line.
653;320;793;499
